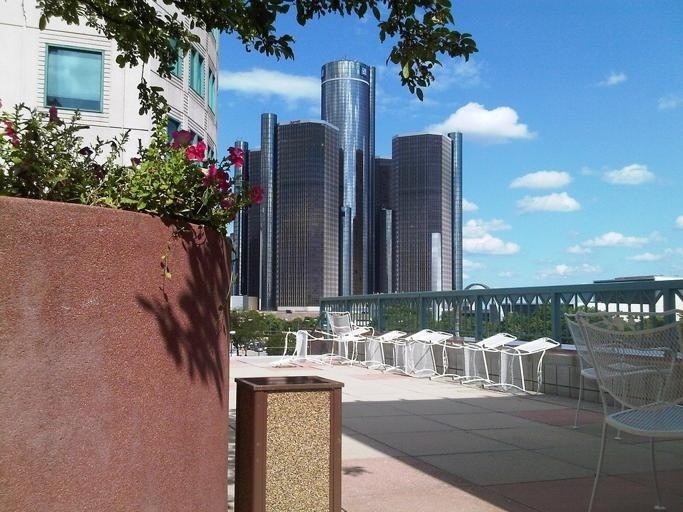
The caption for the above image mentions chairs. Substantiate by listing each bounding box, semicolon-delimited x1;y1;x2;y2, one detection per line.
271;311;561;397
573;307;683;512
564;310;676;442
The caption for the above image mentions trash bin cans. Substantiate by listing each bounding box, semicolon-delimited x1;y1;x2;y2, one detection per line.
235;375;344;512
